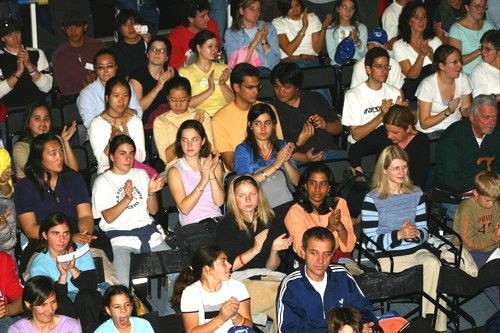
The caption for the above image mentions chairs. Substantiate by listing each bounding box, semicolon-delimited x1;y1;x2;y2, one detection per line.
0;61;500;333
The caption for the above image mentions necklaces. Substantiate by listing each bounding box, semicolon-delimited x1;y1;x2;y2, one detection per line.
106;110;125;118
261;143;272;159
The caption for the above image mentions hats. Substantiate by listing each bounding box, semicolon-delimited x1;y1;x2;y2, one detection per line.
334;37;355;65
62;9;88;25
367;28;387;45
0;18;23;36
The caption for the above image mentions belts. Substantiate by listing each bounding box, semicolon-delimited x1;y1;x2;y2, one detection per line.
280;54;319;62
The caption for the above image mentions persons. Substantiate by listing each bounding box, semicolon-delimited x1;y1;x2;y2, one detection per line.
0;0;500;333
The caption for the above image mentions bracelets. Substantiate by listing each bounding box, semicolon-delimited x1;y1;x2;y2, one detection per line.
336;224;345;232
233;317;245;327
149;193;156;197
299;31;305;36
261;42;268;46
304;115;314;124
12;73;20;80
239;254;245;265
213;317;221;328
29;69;35;75
196;186;202;192
261;170;267;179
0;181;8;185
210;177;216;180
443;109;449;117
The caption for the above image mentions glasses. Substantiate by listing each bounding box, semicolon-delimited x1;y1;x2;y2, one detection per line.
469;3;488;10
479;46;497;52
238;81;263;88
370;63;391;70
389;165;407;172
444;59;463;64
149;46;168;53
